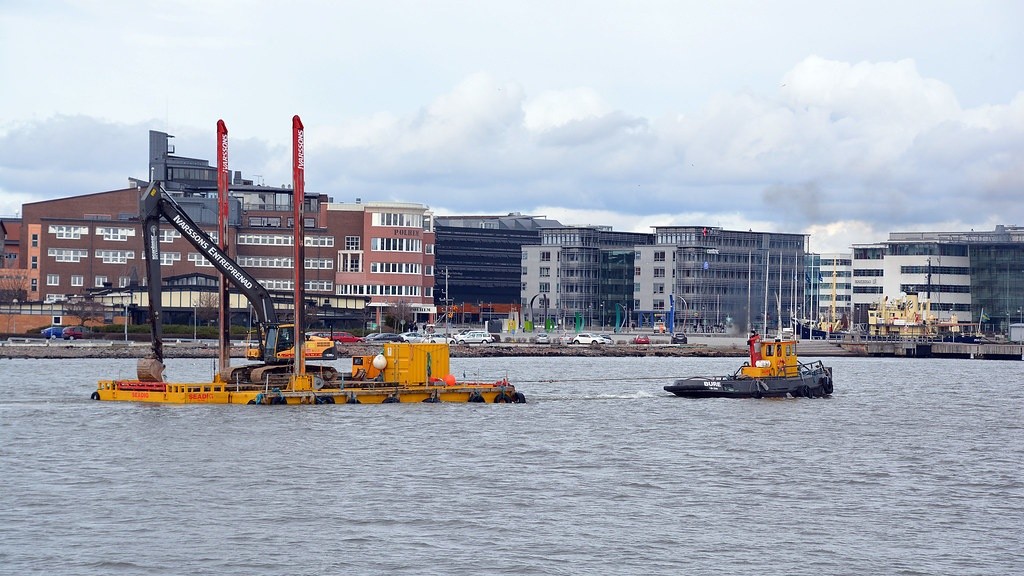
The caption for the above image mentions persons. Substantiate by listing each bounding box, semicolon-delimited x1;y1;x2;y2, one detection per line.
631;322;635;330
414;322;418;332
501;378;506;386
423;322;426;331
812;322;816;329
914;312;918;322
747;330;761;367
819;324;823;331
427;326;435;334
777;346;781;356
368;373;382;382
268;333;289;365
408;321;414;331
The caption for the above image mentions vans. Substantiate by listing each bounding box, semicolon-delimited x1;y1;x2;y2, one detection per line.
653;322;667;332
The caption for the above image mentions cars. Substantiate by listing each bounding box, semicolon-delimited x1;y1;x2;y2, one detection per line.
534;333;551;344
572;333;606;345
632;334;649;344
558;335;574;344
89;112;529;407
599;334;614;344
41;327;65;339
362;332;380;343
671;332;687;343
366;333;404;343
401;332;425;343
421;334;457;345
61;326;94;340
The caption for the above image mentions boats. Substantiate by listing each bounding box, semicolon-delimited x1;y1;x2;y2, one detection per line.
790;254;987;343
662;329;836;400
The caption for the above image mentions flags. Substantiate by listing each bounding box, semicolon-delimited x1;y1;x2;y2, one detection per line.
982;311;990;321
818;271;823;282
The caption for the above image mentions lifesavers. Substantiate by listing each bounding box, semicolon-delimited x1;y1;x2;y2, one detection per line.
777;360;785;371
494;393;512;404
317;395;336;404
423;397;439;402
513;392;526;403
799;386;812;398
823;377;834;393
91;392;100;400
346;399;361;404
270;397;287;405
381;397;400;403
467;395;485;403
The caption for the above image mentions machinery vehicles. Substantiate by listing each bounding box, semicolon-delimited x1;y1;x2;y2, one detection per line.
137;180;340;386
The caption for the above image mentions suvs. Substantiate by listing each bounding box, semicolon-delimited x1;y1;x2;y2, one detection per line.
452;327;495;344
311;331;363;345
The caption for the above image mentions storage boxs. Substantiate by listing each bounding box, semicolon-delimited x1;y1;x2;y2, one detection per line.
381;341;452;385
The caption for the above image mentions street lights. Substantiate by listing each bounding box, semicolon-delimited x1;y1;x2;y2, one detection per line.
12;298;18;334
1004;311;1011;339
1017;305;1022;343
192;302;197;341
123;302;130;342
